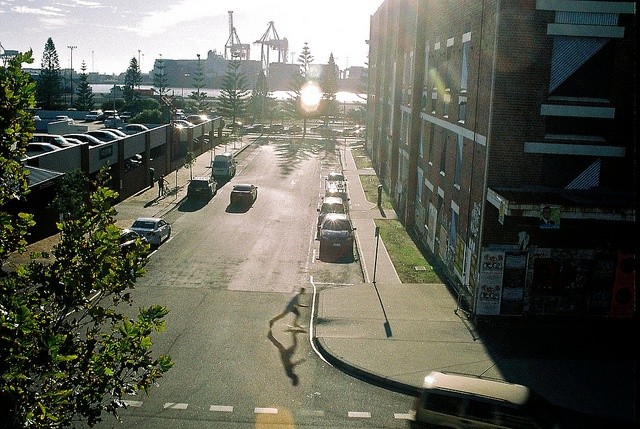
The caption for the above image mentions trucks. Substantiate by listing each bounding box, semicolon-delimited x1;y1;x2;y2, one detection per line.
326;171;347;200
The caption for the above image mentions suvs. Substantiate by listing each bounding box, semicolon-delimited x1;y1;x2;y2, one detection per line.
88;131;119;142
28;143;59;152
187;115;204;123
187;176;218;199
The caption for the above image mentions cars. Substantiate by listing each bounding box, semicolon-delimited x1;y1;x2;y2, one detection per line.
231;184;257;206
63;134;103;145
129;217;171;245
309;125;366;137
246;124;302;135
85;111;101;120
119;228;148;254
119;124;148;133
318;212;356;253
105;116;120;127
226;122;242;129
176;120;192;127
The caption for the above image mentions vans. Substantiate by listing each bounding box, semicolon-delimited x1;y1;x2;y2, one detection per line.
212;152;238;178
416;371;555;428
33;135;76;148
317;196;344;212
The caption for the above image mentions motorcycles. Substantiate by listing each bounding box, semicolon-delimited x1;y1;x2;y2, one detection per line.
163;183;172;198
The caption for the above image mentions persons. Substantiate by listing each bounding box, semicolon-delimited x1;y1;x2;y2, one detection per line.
158;174;169;196
269;287;309;328
